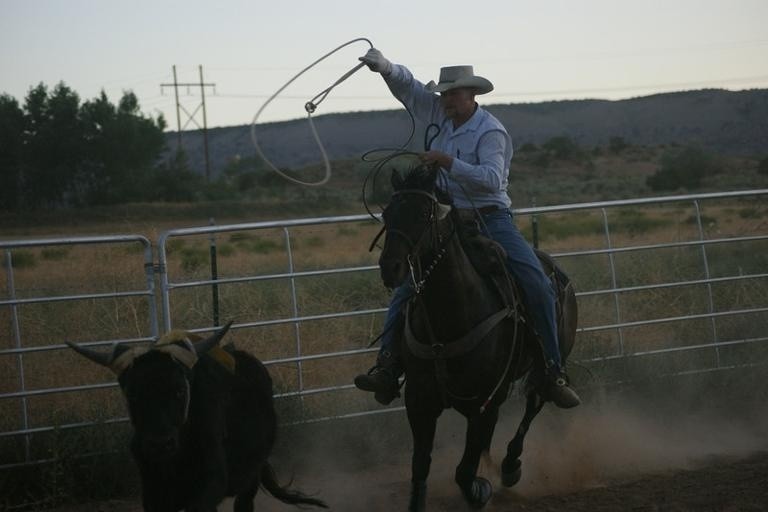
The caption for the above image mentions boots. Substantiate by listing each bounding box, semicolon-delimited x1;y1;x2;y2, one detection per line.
539;374;580;408
355;344;400;405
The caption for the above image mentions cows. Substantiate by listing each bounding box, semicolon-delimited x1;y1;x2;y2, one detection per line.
61;317;332;512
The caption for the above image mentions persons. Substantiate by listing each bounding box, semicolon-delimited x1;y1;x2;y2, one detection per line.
351;47;583;410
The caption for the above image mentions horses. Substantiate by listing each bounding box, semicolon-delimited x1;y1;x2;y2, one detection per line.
375;158;579;512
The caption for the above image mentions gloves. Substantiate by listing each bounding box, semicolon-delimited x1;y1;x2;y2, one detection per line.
359;49;388;72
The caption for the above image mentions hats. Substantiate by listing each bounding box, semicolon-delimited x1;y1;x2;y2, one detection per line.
424;66;493;96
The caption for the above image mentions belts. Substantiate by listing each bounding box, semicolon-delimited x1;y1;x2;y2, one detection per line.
462;206;499;219
